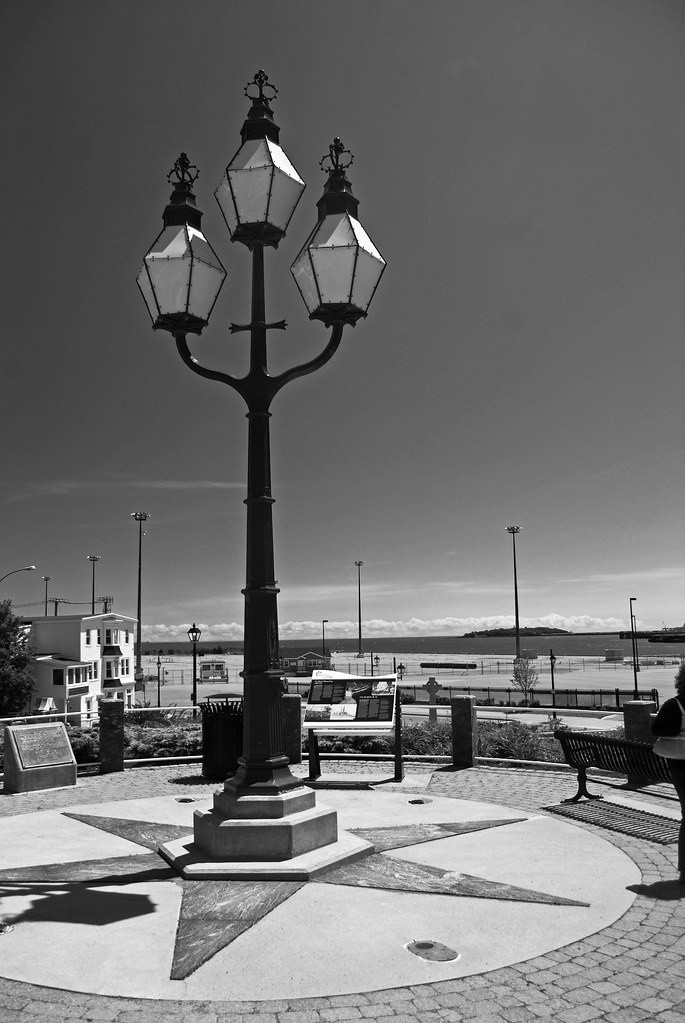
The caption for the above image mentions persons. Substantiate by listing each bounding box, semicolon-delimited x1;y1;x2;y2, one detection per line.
651;662;685;882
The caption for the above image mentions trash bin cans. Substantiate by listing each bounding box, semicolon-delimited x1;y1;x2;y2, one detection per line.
622;701;659;784
197;692;243;780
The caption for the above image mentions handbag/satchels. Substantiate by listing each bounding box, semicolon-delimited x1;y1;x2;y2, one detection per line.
652;737;685;759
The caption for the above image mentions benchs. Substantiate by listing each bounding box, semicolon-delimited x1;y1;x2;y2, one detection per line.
552;729;675;803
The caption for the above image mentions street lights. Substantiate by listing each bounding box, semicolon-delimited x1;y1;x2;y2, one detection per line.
629;597;640;700
632;614;641;672
186;622;202;717
322;619;328;656
550;653;557;719
41;576;52;617
505;526;522;664
353;560;366;658
156;661;162;707
86;554;101;614
130;511;151;682
